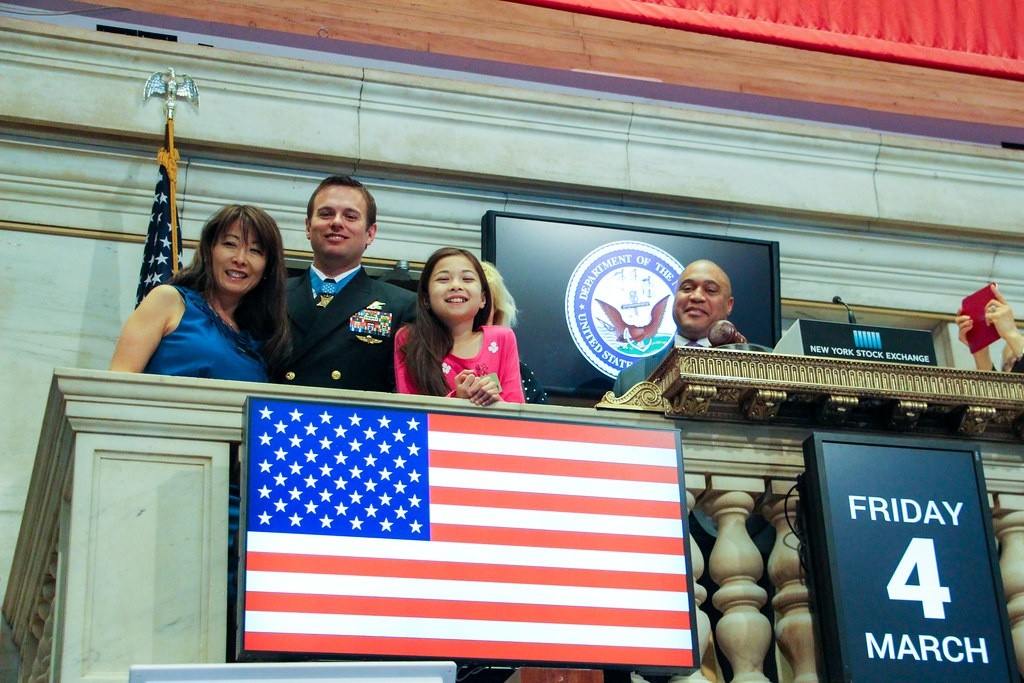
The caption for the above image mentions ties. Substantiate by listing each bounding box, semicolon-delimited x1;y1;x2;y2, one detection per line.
314;278;336;311
686;340;700;346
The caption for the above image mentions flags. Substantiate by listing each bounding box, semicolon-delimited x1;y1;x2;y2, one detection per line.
135;130;181;307
243;397;694;667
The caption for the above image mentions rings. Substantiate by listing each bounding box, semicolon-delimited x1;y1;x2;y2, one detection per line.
991;306;996;312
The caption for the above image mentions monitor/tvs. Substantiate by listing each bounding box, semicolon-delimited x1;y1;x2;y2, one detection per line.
481;210;780;400
803;432;1023;683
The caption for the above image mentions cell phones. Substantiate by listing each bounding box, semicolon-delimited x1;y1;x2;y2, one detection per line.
962;283;1001;354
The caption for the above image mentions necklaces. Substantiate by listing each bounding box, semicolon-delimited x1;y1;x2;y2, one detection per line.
229;322;239;327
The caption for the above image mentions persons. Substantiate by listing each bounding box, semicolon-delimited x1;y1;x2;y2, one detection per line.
613;259;777;682
264;176;418;392
108;205;291;381
394;247;551;407
956;285;1024;372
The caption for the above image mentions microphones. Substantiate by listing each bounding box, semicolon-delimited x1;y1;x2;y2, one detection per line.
833;296;856;324
